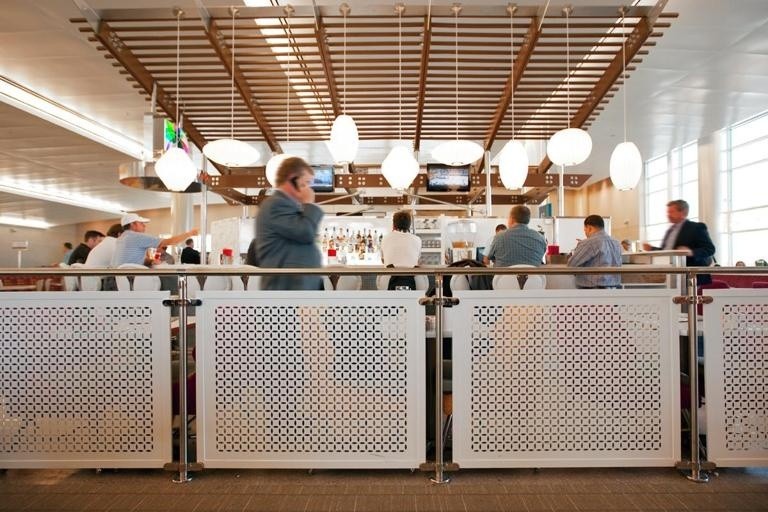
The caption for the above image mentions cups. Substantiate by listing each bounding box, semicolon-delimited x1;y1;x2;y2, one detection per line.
421;239;440;247
155;253;161;264
755;258;763;267
173;247;183;264
628;239;639;254
547;245;560;255
148;247;155;260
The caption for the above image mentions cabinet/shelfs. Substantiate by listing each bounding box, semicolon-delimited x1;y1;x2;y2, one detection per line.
416;213;445;264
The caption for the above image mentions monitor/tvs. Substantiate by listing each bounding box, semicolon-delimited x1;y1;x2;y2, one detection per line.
310;164;335;192
426;162;471;192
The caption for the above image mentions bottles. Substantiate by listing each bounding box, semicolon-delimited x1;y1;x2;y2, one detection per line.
322;226;385;254
416;219;439;229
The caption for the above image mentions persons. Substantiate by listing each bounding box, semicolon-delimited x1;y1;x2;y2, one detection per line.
68;229;107;267
180;238;200;265
243;155;326;289
379;212;424;290
619;237;631;256
159;246;177;264
481;222;507;267
50;241;75;267
111;211;201;268
567;214;623;290
641;197;716;287
468;206;547;291
84;224;123;268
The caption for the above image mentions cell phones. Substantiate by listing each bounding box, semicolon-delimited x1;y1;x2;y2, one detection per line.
291;176;299;187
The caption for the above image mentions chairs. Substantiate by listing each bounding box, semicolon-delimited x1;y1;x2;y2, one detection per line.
0;259;767;336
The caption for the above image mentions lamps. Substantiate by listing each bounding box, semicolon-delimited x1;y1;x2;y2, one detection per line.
265;4;305;189
546;6;593;165
328;2;359;166
381;3;420;191
498;3;529;191
430;3;485;168
154;6;199;193
202;7;262;168
609;6;643;191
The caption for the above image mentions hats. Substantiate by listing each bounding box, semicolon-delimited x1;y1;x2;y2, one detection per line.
120;213;150;227
